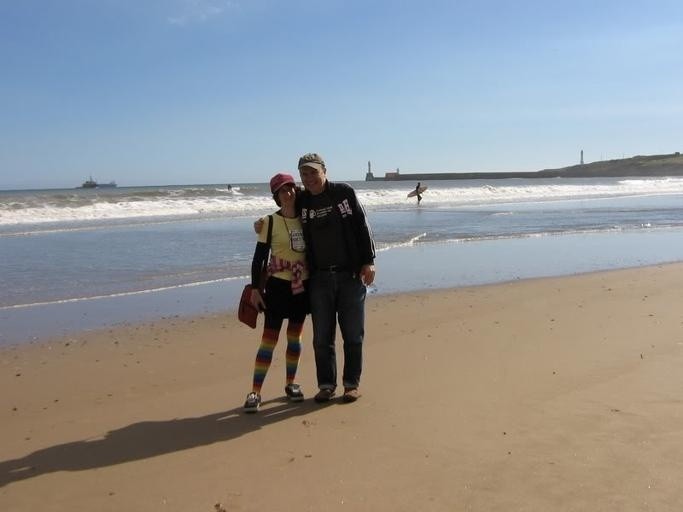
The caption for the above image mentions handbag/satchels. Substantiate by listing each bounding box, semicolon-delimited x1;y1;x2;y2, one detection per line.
237;282;268;329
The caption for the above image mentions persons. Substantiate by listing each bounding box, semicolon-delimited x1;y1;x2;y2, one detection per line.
253;152;376;403
241;172;309;413
415;180;422;205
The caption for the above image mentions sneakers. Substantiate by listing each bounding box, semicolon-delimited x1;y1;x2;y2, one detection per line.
343;386;362;401
284;381;305;401
242;391;261;412
314;386;337;403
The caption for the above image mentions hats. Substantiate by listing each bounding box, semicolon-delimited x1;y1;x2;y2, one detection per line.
297;152;325;172
269;172;296;195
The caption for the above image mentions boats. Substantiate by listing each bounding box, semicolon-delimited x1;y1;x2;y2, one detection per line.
74;174;116;190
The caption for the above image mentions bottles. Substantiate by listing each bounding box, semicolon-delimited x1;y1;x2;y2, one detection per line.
361;271;377;295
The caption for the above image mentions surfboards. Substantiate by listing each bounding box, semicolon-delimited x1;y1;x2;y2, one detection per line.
408;187;427;197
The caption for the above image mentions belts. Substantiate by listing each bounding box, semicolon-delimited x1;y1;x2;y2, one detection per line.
307;261;359;273
